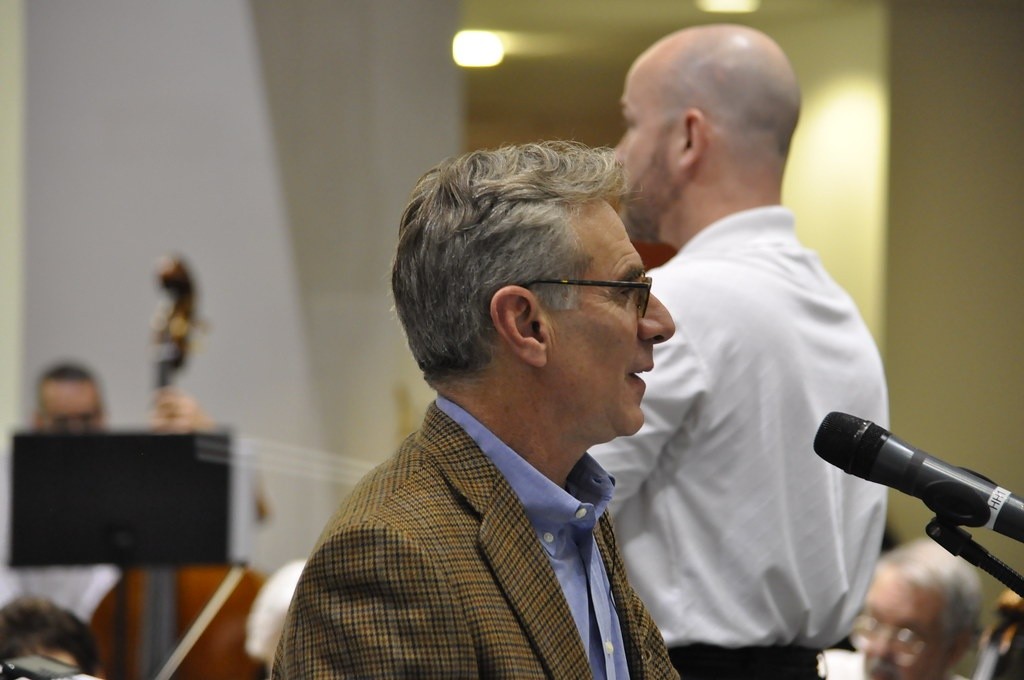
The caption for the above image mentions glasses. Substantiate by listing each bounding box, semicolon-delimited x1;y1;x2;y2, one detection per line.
852;615;925;657
484;273;654;324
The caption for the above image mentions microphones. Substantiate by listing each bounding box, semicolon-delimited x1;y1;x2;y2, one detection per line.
814;411;1024;543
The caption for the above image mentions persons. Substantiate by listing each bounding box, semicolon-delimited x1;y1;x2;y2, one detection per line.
1;357;208;680
817;535;987;680
585;24;890;680
272;142;682;680
